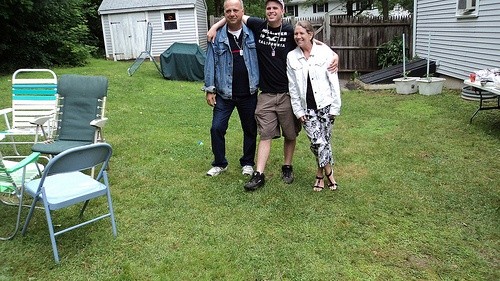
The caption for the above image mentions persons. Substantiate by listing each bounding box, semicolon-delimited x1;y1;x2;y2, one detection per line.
286;21;343;191
207;0;339;192
203;0;258;177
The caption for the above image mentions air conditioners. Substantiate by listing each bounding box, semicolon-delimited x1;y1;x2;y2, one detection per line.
456;0;476;15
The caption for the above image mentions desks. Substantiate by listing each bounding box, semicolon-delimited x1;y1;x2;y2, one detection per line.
464;79;500;124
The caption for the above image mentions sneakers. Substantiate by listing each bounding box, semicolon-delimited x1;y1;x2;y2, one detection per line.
242;165;254;175
207;167;227;176
282;166;294;184
244;171;265;190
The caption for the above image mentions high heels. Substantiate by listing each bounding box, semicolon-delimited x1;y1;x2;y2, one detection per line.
313;176;325;191
325;168;338;190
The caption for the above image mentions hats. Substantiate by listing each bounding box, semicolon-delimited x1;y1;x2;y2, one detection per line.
265;0;285;9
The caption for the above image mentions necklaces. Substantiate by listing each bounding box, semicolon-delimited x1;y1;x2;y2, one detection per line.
233;34;244;55
266;22;282;56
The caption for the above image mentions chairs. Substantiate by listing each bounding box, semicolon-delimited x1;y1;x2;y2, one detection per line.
0;69;117;264
